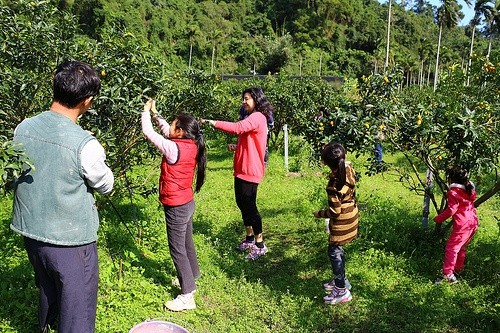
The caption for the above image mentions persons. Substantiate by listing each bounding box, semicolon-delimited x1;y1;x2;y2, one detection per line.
141;97;208;311
433;166;478;286
10;60;114;333
198;87;275;261
375;130;384;171
312;143;358;304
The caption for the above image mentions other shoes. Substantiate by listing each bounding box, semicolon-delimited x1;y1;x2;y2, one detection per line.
436;275;457;285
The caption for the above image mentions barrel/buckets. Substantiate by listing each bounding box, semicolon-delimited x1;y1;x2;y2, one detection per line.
127;316;195;333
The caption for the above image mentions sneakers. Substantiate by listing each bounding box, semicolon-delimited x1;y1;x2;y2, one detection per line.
164;295;196;311
237;239;255;251
246;245;269;261
323;278;352;291
324;286;353;305
